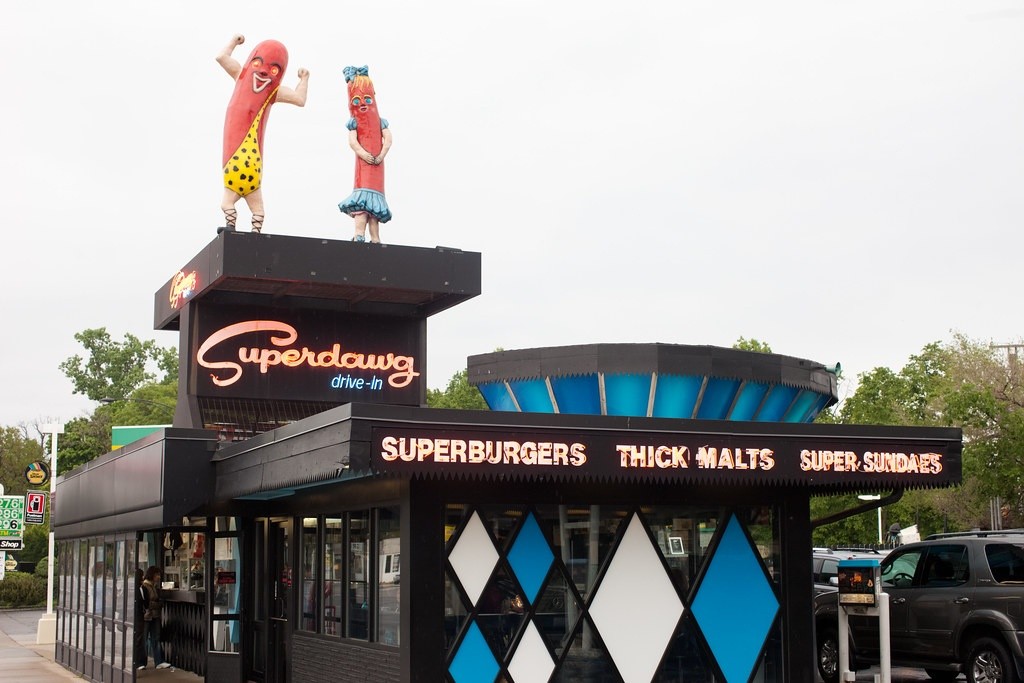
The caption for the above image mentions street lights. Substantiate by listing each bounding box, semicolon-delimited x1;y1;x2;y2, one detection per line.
40;423;65;612
857;495;884;547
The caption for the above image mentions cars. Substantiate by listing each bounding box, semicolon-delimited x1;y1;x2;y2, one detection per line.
871;549;920;566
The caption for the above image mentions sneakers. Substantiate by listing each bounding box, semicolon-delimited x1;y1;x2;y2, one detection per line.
156;662;171;669
136;665;147;670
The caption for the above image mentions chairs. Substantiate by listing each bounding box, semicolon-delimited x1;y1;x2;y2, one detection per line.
989;560;1011;583
926;559;955;588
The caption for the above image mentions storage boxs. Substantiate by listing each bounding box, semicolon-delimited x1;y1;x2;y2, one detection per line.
670;516;700;552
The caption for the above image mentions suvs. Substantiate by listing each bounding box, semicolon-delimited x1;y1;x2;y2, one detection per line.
814;528;1024;683
812;547;920;597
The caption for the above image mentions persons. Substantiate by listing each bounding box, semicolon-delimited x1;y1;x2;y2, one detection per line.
214;567;233;606
143;566;171;669
767;565;773;578
134;569;148;669
481;574;523;635
